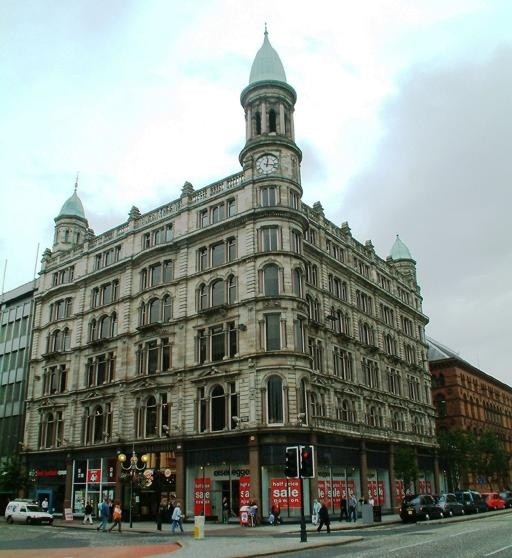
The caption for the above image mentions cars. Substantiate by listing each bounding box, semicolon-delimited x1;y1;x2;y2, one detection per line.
4;501;53;525
400;489;512;522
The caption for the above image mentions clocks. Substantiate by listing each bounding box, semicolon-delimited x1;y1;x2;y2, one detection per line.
255;153;280;175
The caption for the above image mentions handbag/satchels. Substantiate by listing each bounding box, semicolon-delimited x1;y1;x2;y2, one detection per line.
98;502;103;510
268;514;275;524
113;512;121;521
172;512;179;521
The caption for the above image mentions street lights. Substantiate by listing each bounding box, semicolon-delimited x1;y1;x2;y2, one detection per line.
118;453;148;528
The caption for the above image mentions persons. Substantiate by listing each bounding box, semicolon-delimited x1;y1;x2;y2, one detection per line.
165;501;174;522
108;501;122;532
82;501;94;525
339;495;351;522
368;496;374;507
223;496;230;524
316;502;331;533
348;493;357;522
170;502;186;533
96;496;110;530
41;497;49;512
156;497;169;531
312;498;323;524
271;500;280;526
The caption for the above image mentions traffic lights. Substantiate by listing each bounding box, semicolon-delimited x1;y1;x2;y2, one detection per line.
284;446;313;477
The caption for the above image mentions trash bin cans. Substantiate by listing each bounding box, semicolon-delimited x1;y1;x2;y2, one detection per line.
373;506;381;522
